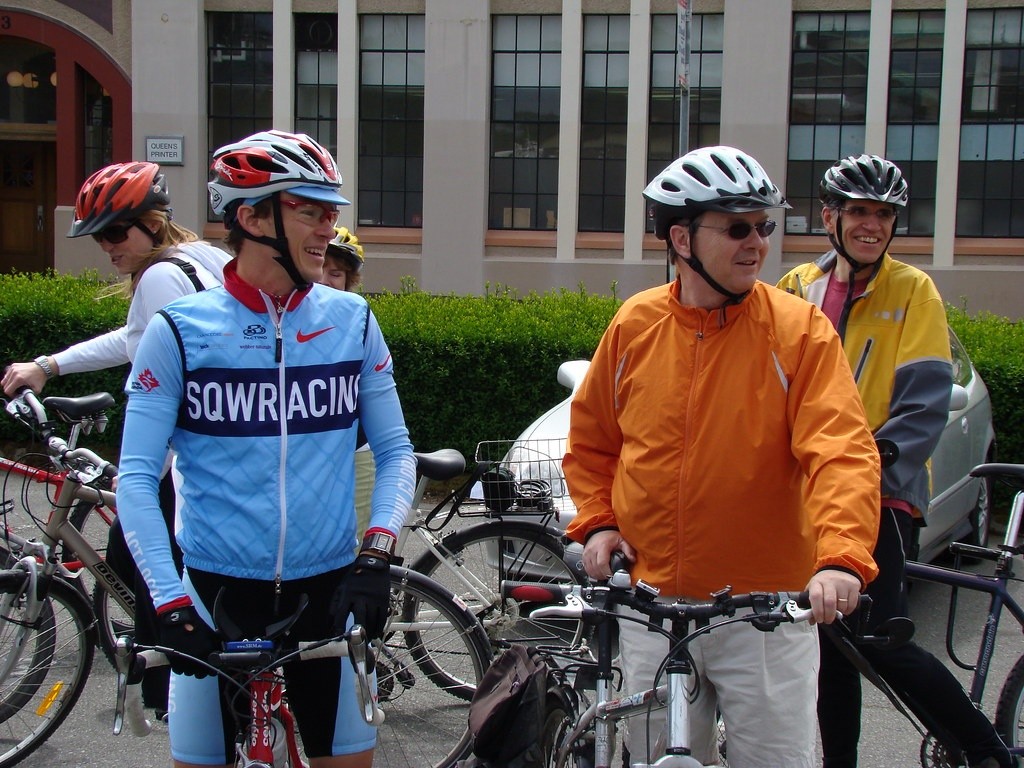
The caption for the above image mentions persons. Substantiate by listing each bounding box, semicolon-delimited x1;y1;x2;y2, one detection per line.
560;146;880;768
117;127;418;768
770;152;954;768
0;155;364;508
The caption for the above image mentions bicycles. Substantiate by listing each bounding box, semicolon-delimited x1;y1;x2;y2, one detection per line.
467;540;917;767
0;366;592;768
875;437;1023;768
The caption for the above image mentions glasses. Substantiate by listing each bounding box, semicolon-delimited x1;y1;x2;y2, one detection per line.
832;205;900;220
259;199;341;226
91;222;134;244
324;265;348;277
687;220;778;240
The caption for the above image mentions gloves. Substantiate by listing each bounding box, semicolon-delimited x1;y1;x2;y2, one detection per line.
153;605;224;679
340;554;390;644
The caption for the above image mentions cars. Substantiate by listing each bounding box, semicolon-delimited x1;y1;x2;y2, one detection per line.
470;325;994;584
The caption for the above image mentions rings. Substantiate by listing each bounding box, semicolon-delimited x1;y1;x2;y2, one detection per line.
837;598;848;602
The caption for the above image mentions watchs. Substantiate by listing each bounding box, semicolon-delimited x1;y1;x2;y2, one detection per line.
34;355;54;378
359;530;397;560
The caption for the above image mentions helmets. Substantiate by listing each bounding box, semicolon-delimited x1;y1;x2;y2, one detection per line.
817;154;908;207
206;129;351;215
329;227;365;272
66;161;172;238
641;145;794;240
